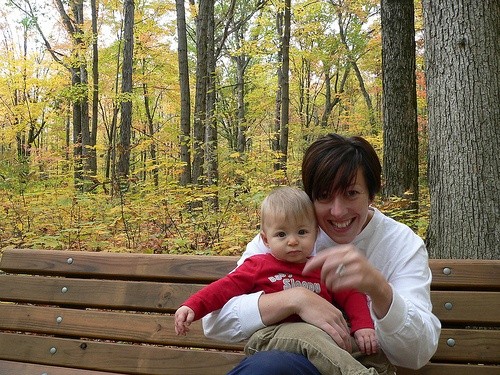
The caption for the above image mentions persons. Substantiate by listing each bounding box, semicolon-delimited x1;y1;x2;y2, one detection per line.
201;134;441;375
175;188;400;375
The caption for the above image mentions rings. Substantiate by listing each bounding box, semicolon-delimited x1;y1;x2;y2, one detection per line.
334;264;347;276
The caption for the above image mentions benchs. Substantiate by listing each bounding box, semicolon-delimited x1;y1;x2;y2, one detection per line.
0;249;500;375
